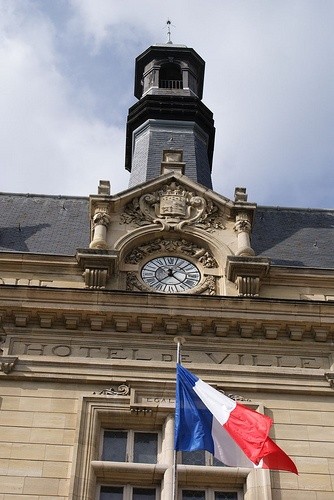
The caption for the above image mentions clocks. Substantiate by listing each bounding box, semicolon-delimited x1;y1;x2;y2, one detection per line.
139;255;202;293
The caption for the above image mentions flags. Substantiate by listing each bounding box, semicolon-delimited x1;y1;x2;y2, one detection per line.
174;361;300;475
